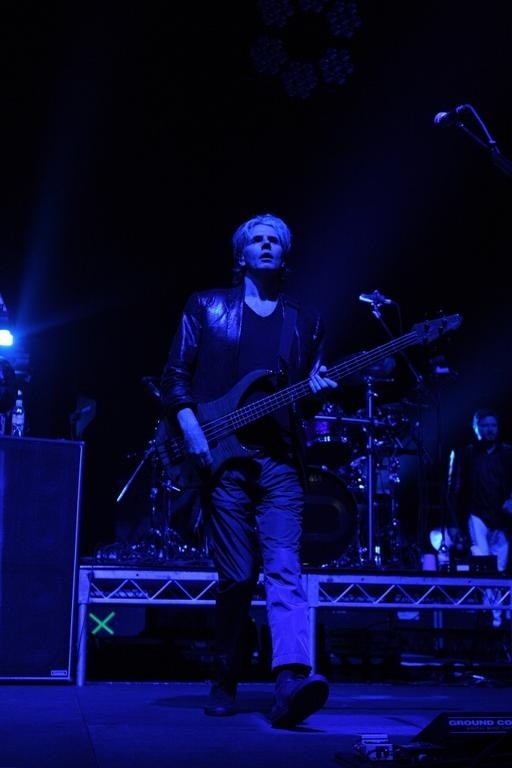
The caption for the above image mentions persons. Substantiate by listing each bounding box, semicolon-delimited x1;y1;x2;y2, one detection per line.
160;212;338;729
448;409;512;627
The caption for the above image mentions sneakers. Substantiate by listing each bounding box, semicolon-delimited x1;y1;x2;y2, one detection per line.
204;685;238;717
272;674;329;728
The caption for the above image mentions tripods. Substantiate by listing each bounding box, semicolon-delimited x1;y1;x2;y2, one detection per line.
98;376;406;570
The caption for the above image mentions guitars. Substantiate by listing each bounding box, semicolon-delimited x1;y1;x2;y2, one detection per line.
127;311;462;513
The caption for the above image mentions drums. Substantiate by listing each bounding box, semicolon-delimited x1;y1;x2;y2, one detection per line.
304;414;344;450
298;465;358;566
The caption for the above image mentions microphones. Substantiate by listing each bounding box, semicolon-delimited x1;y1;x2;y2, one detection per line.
433;105;465;129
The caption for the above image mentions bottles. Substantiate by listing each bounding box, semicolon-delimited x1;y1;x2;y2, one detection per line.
11;399;25;437
438;540;451;572
